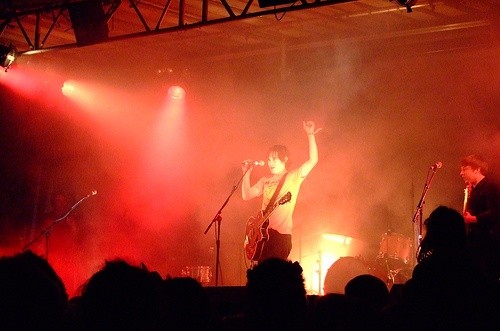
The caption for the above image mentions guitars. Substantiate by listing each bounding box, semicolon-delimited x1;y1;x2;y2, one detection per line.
244;191;292;270
461;180;471;218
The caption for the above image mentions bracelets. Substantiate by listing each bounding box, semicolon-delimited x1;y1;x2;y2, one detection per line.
307;132;315;135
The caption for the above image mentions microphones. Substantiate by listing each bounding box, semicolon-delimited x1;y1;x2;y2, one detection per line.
244;161;264;166
86;190;97;198
430;162;442;169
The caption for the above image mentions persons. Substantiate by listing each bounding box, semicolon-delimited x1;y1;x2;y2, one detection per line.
0;205;500;331
241;121;318;264
460;153;500;249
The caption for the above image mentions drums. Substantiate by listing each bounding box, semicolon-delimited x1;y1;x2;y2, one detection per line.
180;264;212;283
323;255;385;296
377;232;414;266
